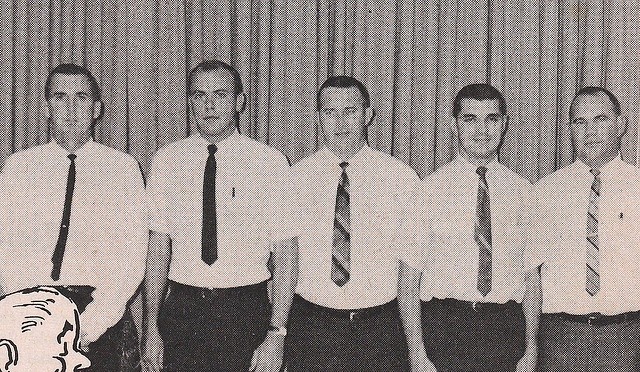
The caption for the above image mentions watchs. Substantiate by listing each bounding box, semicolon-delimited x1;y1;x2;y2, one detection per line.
267;326;288;336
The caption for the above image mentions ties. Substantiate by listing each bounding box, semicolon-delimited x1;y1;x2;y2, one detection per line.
474;167;492;297
331;162;350;288
201;145;217;267
586;170;601;297
51;154;77;281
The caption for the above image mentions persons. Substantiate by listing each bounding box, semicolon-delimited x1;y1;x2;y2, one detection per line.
0;63;149;372
140;60;301;372
397;84;546;372
534;87;640;372
267;76;421;372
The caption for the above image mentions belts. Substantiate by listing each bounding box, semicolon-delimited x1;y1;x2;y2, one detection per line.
295;294;397;321
543;311;639;326
422;300;522;312
168;280;268;304
